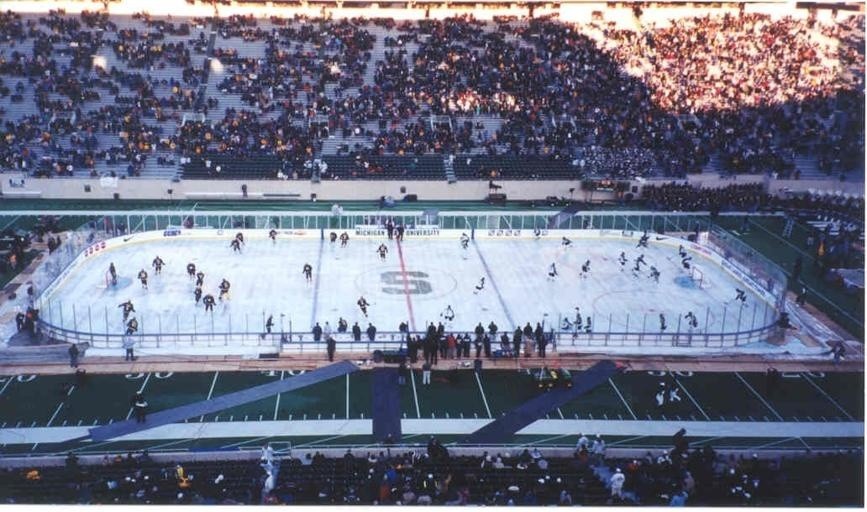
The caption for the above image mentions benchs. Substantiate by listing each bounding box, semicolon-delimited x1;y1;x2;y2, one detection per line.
0;0;864;178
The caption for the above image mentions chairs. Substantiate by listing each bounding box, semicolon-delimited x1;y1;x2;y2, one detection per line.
1;462;604;504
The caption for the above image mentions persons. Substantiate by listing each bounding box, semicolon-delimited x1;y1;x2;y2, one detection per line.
458;233;470;248
195;270;204;286
187;263;196;279
632;254;646;272
356;295;369;317
137;268;148;290
387;222;394;239
2;1;863;230
375;243;388;261
396;224;404;241
646;266;660;282
235;231;245;246
338;231;349;248
218;278;230;299
228;238;243;255
301;261;312;283
108;261;117;285
578;259;591;278
620;251;627;269
677;244;686;257
561;236;574;250
269;229;277;240
329;231;337;243
1;381;864;506
14;305;847;386
3;222;130;279
193;286;203;303
151;255;166;275
635;235;650;248
201;294;216;311
117;300;136;321
730;223;864;305
472;276;485;295
545;262;559;282
